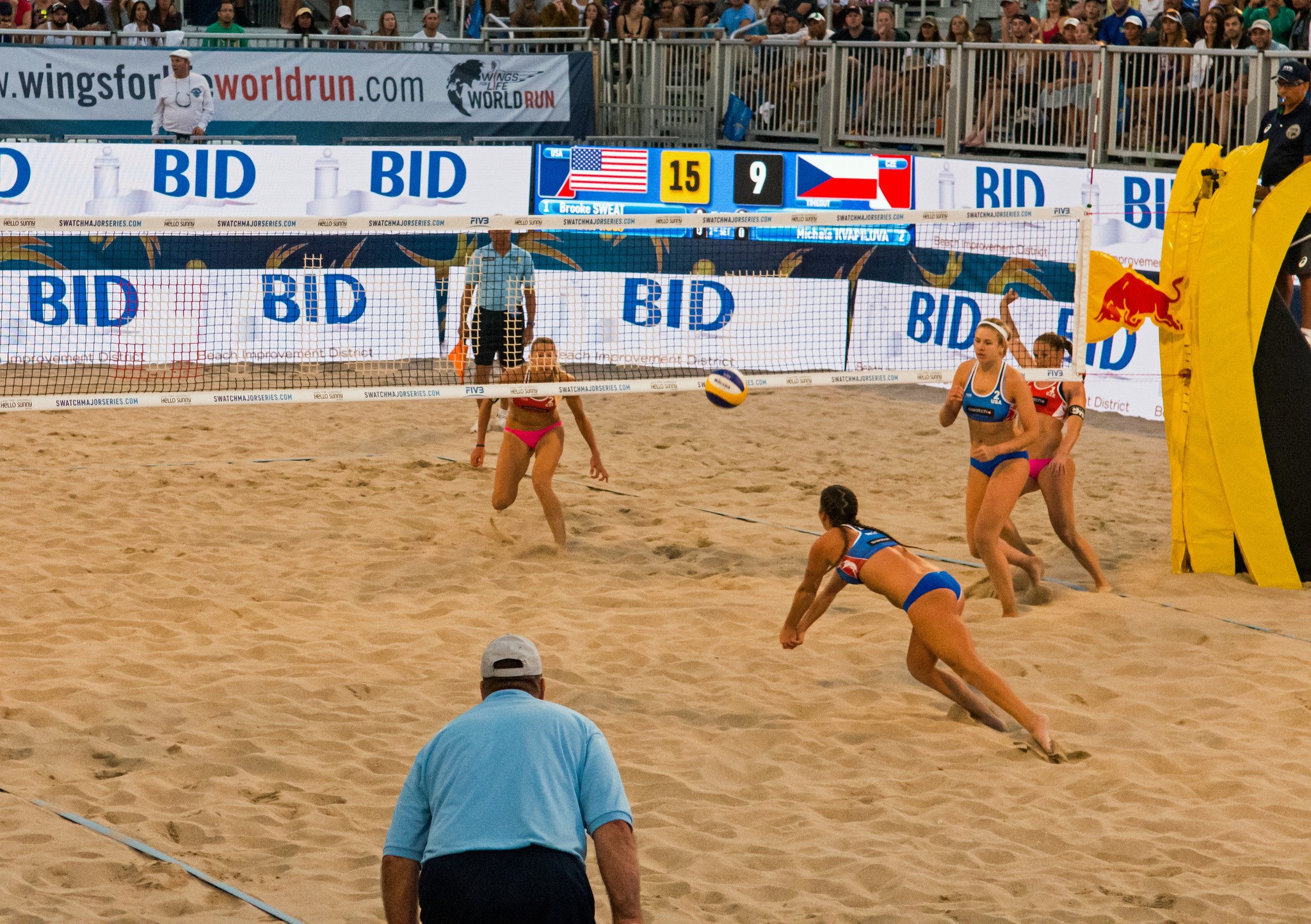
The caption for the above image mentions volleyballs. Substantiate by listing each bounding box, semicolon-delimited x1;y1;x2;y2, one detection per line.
705;366;748;411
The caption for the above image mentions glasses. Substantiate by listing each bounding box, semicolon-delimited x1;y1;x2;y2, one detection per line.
1162;10;1180;19
1274;80;1306;88
1065;26;1075;30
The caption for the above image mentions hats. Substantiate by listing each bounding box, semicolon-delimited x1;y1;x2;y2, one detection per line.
479;633;543;678
808;12;826;22
788;10;803;22
335;5;352;18
1012;13;1032;32
1000;0;1019;7
1270;60;1310;83
296;7;312;17
846;5;863;15
1123;15;1142;27
1160;8;1183;24
421;7;439;22
1064;17;1081;28
1249;19;1272;32
52;2;67;12
170;49;192;60
771;5;786;14
921;16;938;27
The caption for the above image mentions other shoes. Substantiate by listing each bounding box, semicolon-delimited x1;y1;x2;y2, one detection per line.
1150;139;1186;154
960;136;985;148
779;119;811;132
846;130;862;146
1127;124;1152;147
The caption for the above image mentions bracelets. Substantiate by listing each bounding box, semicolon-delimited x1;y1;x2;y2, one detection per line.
476;444;485;447
1268;184;1275;191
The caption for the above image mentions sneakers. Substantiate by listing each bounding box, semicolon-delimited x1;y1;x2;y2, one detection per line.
495;412;508;429
470;419;491;434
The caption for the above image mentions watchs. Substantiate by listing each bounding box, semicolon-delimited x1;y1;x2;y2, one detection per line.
527;321;535;326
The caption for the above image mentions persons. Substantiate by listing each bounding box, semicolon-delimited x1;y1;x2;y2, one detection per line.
369;11;403;51
0;0;367;49
410;7;451;52
938;316;1044;618
381;634;645;924
996;288;1110;593
1252;60;1311;346
471;337;610;547
457;213;537;432
779;483;1052;753
457;0;1311;158
151;49;214;144
